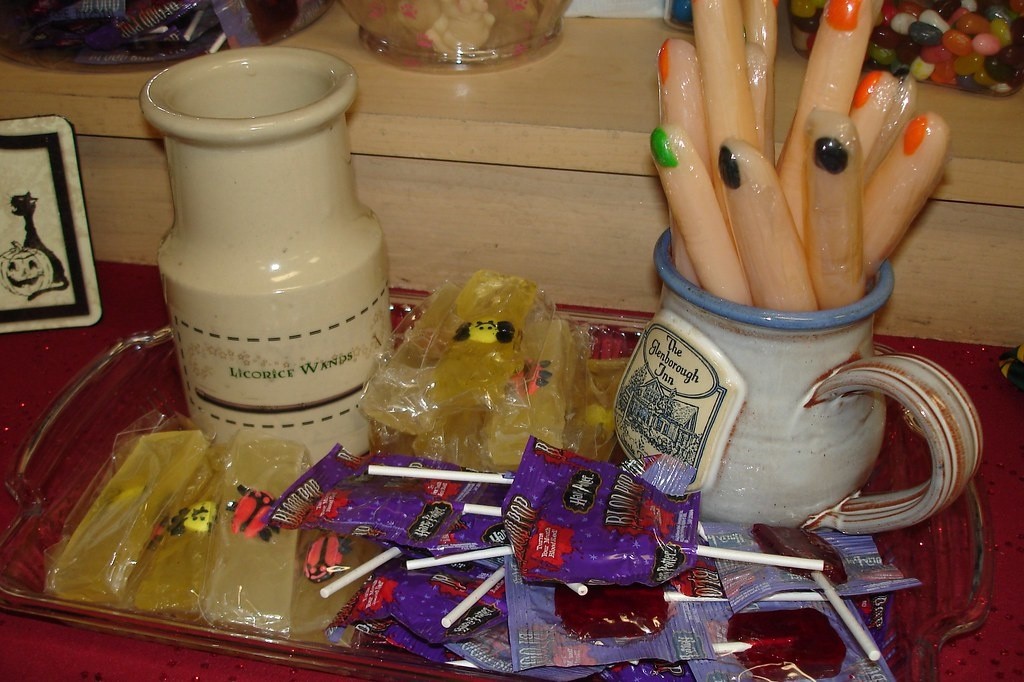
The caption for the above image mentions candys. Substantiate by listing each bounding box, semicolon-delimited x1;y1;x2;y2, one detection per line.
790;0;1024;99
42;270;922;679
0;0;331;72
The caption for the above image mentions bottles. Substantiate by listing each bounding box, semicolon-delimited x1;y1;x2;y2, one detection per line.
339;0;573;76
140;47;394;468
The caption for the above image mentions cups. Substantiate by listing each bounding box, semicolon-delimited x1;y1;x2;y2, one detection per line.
613;229;982;535
